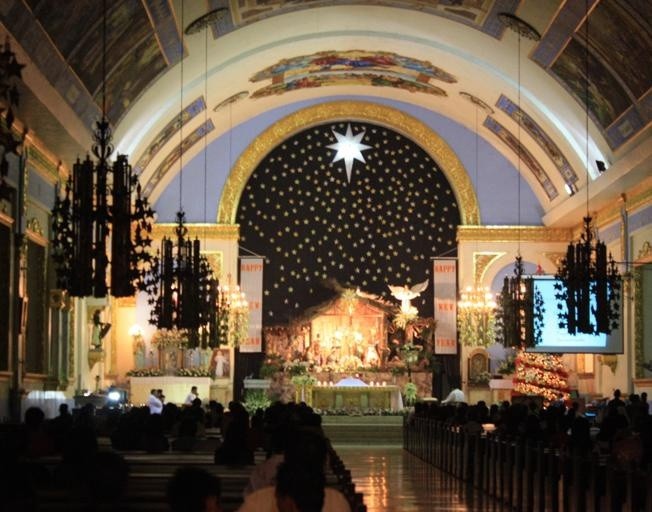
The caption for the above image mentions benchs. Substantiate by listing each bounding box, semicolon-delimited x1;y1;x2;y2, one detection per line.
45;427;367;512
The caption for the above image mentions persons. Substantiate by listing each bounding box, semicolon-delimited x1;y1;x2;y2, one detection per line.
406;383;652;474
